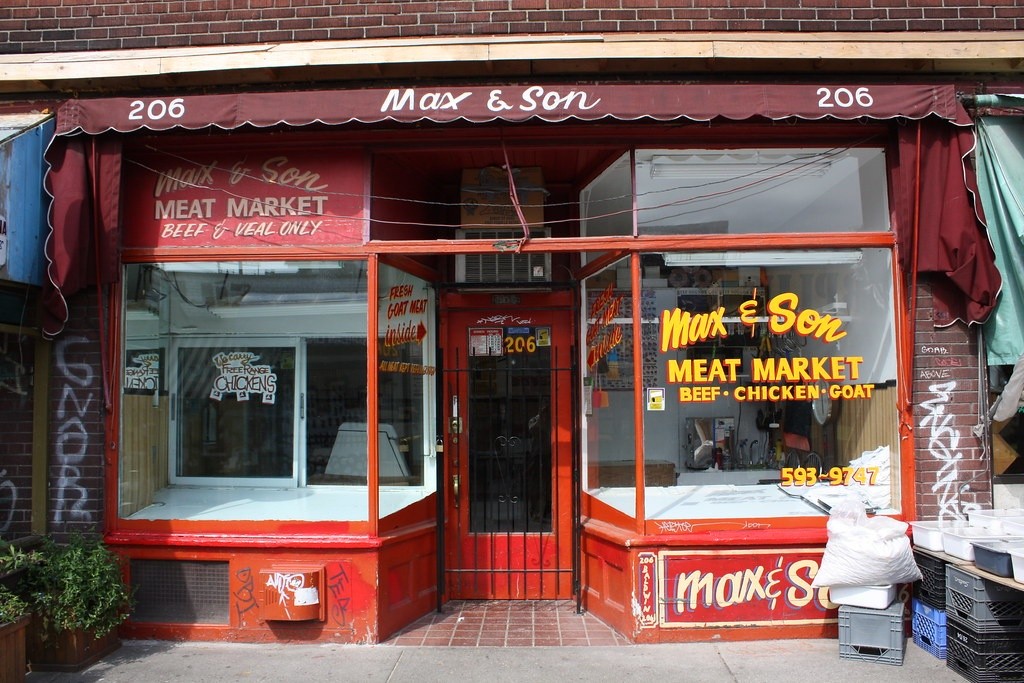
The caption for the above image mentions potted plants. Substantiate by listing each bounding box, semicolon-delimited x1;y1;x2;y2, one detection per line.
0;533;132;683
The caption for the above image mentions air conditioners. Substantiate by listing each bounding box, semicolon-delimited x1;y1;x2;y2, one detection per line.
455;229;554;293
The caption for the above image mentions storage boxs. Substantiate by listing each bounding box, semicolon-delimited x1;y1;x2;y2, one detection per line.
462;167;545;230
829;518;1024;683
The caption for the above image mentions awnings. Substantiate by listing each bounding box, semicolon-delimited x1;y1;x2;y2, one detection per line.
39;84;1003;343
974;91;1024;366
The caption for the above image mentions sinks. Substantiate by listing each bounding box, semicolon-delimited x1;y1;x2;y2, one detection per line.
724;466;771;471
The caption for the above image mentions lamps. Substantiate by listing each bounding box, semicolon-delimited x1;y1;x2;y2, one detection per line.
158;262;345;274
662;249;861;266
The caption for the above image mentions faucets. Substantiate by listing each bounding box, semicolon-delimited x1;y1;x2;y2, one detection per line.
749;440;758;461
